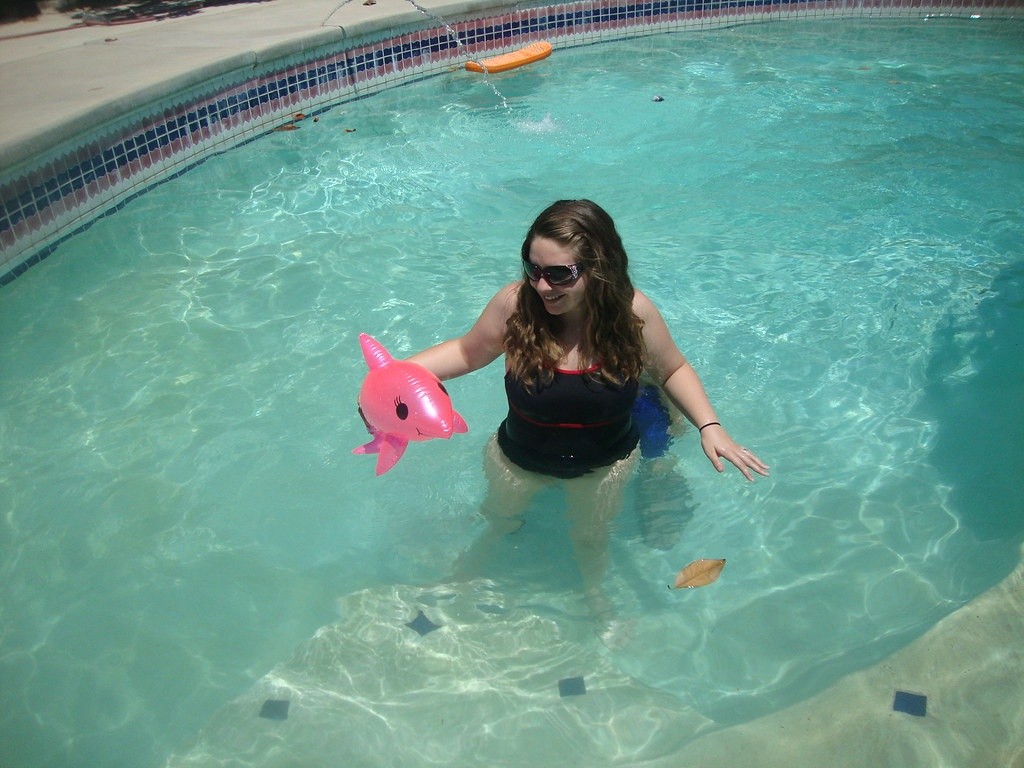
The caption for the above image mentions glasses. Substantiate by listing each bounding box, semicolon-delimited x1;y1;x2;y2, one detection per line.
523;260;583;285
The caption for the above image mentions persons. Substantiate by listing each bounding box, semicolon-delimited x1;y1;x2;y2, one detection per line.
356;197;773;485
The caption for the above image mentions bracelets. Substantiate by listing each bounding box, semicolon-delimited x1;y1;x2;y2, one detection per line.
699;421;722;435
